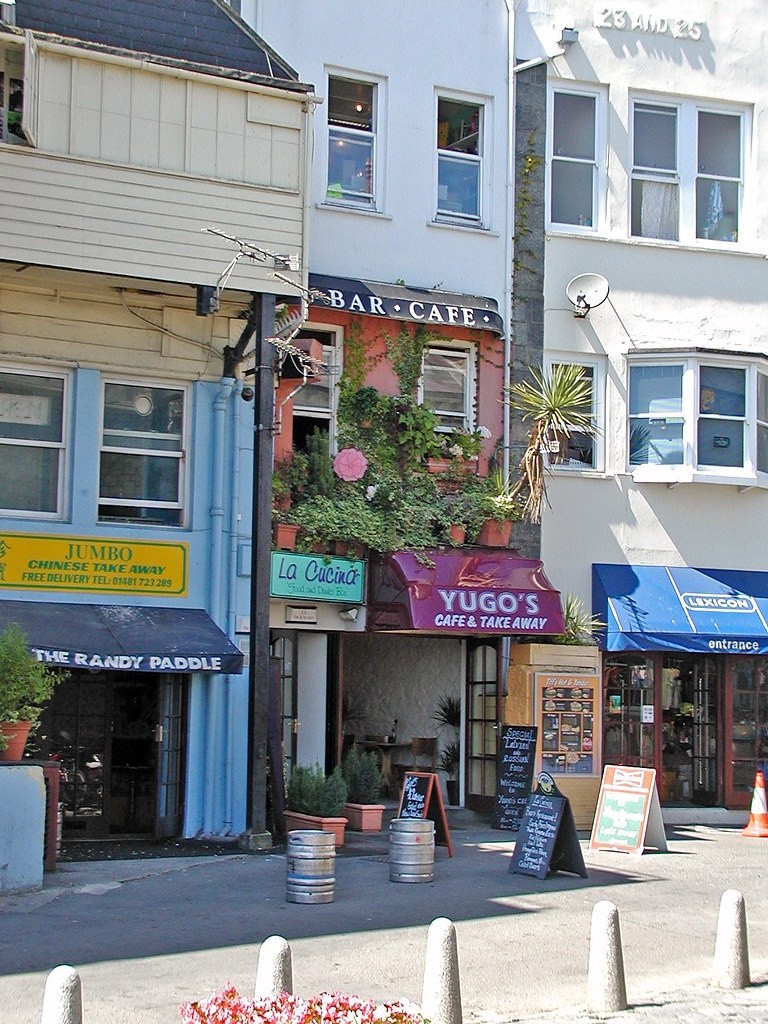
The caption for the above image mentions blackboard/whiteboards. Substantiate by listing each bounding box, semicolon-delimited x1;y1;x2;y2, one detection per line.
507;794;587;875
494;726;538;831
398;772;452;846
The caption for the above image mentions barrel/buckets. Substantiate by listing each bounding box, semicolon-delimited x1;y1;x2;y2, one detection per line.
285;829;337;905
388;818;436;883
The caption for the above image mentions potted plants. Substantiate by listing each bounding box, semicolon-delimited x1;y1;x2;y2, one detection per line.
284;759;349;847
344;746;386;833
271;362;597;570
0;620;73;761
430;693;461;806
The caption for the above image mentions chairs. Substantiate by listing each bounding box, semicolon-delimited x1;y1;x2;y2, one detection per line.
395;737;437;802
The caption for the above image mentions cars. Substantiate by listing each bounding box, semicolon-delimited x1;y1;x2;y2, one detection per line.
704;713;768;744
604;706;659;769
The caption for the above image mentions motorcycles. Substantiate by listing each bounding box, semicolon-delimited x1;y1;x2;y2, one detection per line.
23;732;105;804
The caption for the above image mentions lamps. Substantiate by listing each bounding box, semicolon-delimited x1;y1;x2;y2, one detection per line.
197;285;217;318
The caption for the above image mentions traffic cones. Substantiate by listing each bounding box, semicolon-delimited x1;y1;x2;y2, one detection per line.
739;770;768;838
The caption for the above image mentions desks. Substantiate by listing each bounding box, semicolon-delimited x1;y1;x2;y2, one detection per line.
357;742;413;797
110;763;151;832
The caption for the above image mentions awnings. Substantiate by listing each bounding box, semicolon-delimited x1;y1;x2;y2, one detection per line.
369;552;565;635
591;564;768;654
0;600;243;675
